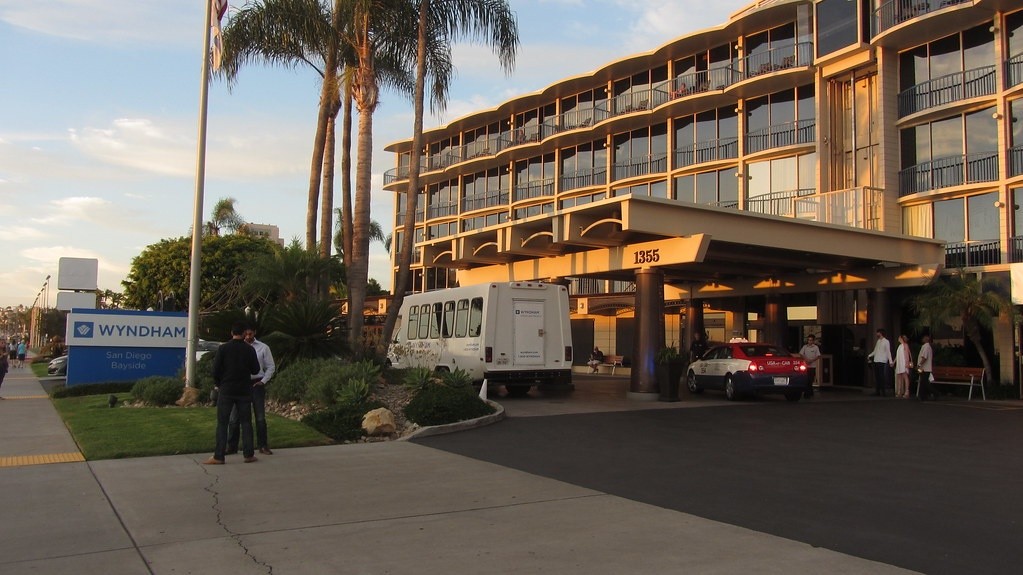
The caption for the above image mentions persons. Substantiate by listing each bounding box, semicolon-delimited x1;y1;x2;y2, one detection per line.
203;321;276;464
590;346;604;373
517;130;525;140
799;334;821;400
726;348;731;358
690;332;705;363
0;337;29;388
679;82;687;94
867;328;933;401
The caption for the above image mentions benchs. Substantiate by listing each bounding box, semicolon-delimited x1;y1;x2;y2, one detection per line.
912;367;985;402
587;356;624;375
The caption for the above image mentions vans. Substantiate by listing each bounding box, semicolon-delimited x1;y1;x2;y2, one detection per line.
388;281;574;395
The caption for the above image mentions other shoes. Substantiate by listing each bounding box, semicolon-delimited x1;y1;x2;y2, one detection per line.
245;455;258;463
0;397;7;400
260;447;272;455
896;394;910;400
224;448;237;455
204;457;224;465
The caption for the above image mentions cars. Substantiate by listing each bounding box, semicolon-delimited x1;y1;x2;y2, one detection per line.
47;353;68;377
196;341;226;362
686;337;808;402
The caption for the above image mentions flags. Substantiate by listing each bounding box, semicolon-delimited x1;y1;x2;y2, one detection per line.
210;0;227;75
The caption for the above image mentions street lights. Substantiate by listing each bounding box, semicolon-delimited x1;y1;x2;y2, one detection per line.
30;287;44;349
46;275;51;313
43;282;47;313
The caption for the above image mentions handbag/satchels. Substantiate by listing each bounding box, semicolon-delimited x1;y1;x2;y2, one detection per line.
906;362;913;369
